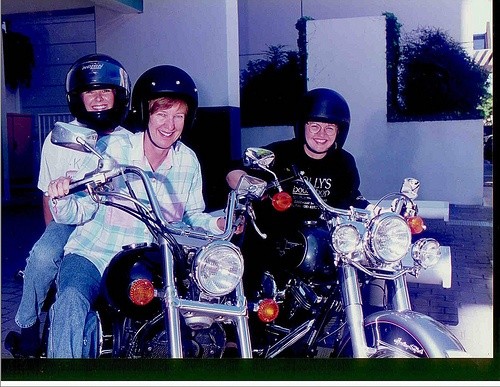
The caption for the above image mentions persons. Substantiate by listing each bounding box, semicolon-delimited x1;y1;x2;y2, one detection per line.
48;65;243;358
225;89;394;289
14;53;130;351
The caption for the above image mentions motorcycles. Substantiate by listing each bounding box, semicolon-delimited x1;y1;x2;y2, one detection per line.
43;122;281;359
242;147;468;358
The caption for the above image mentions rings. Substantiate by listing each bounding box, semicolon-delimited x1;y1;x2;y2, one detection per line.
50;181;55;184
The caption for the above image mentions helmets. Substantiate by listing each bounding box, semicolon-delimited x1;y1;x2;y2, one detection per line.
294;88;350;148
65;53;132;127
131;65;199;130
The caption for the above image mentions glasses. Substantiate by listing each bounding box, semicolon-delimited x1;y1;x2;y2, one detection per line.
307;122;338;136
